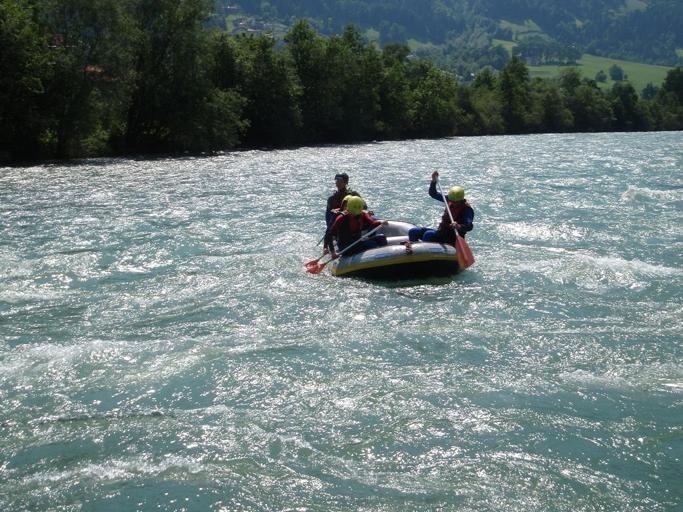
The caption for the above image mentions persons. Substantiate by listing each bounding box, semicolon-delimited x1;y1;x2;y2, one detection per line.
325;196;388;260
407;170;474;245
322;194;374;256
325;172;368;226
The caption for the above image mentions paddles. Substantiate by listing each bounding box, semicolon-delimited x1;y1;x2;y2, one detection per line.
306;224;383;273
304;250;330;267
435;175;475;270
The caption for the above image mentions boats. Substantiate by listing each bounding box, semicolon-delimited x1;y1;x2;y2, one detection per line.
323;219;459;281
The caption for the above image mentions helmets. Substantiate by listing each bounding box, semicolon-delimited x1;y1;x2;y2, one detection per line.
340;195;365;217
448;185;464;202
334;173;348;184
